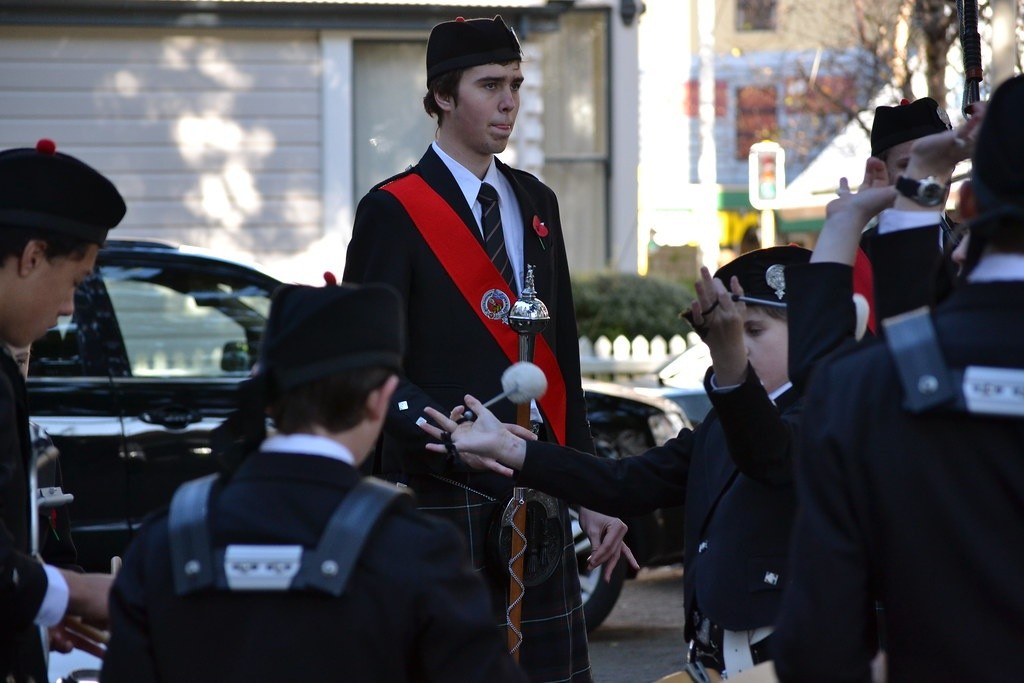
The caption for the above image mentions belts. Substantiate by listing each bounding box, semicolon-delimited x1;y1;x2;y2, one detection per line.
723;626;775;679
529;422;545;434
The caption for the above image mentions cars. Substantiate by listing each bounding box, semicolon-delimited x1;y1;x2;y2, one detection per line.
617;331;740;573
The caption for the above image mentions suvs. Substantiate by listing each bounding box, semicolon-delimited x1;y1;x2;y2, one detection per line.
24;233;700;639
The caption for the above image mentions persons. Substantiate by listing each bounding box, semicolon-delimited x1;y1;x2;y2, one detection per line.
339;12;641;682
98;271;528;683
788;71;1024;683
0;138;116;682
416;242;877;683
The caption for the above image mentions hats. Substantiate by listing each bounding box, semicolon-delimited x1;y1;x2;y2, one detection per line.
0;138;127;245
257;271;406;393
972;74;1024;208
870;97;952;157
426;14;520;90
713;242;813;306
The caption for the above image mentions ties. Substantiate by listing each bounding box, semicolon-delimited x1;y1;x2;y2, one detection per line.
477;182;518;298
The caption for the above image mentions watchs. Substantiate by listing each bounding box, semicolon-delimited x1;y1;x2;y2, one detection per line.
895;175;946;208
